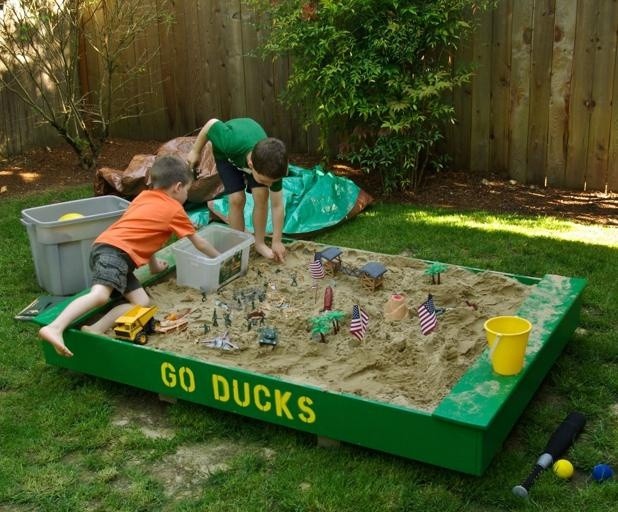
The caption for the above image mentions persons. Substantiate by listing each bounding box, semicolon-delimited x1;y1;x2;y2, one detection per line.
186;116;288;263
36;156;223;360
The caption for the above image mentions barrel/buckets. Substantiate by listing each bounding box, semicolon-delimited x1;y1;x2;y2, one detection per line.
483;315;533;375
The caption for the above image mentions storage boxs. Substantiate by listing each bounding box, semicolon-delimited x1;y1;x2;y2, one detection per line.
170;221;256;291
20;194;131;296
